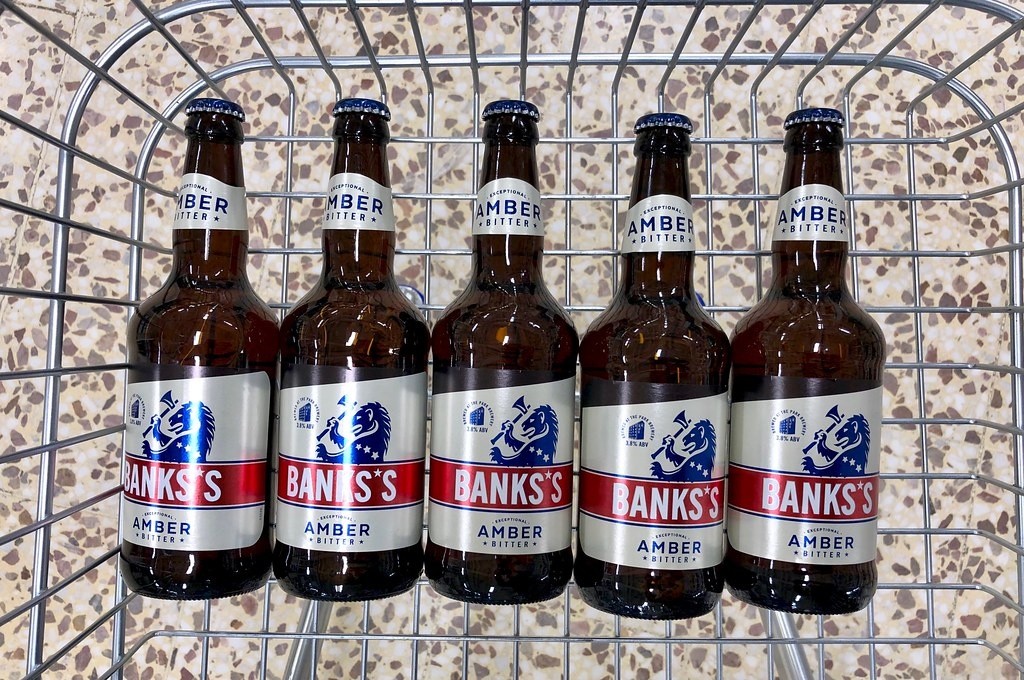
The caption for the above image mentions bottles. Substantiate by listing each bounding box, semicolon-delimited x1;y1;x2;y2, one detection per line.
579;113;733;621
269;99;432;603
119;98;280;601
432;99;580;606
731;108;886;617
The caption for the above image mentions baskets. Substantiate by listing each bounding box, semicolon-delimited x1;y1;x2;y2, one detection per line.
1;0;1024;680
